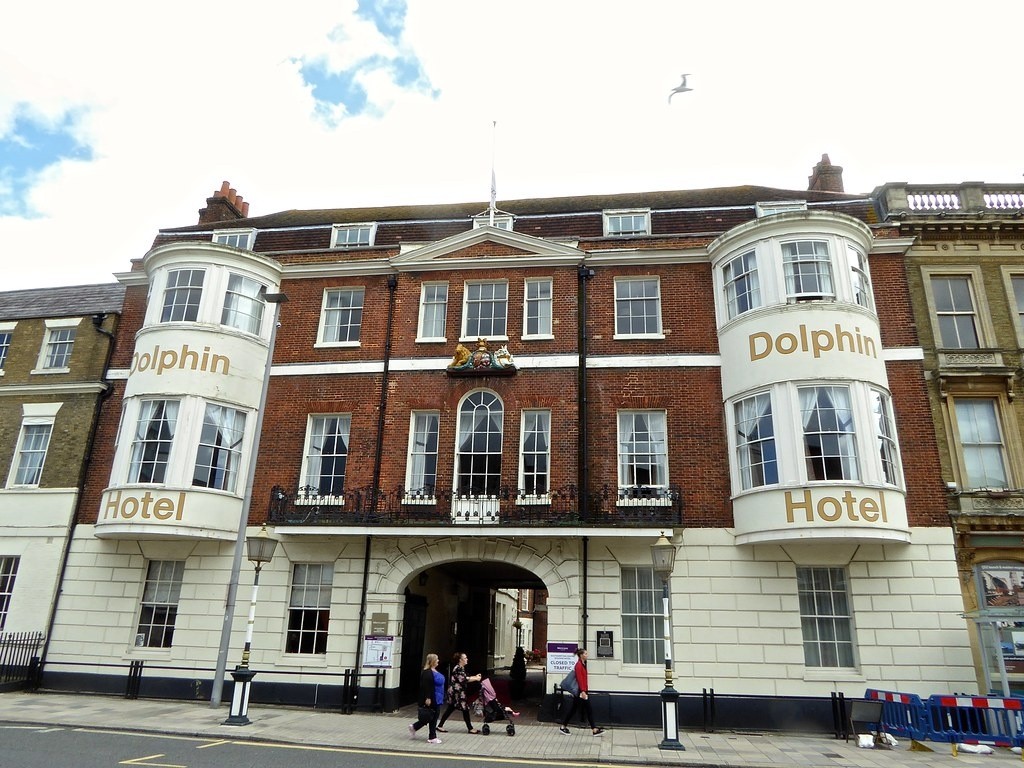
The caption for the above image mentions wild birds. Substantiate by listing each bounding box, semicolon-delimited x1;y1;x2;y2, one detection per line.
669;74;694;102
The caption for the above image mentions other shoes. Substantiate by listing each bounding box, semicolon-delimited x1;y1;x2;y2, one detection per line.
468;728;481;734
436;727;448;732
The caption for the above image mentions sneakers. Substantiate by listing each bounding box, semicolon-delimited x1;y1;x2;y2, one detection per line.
409;724;416;739
560;727;571;735
593;730;606;737
428;738;442;744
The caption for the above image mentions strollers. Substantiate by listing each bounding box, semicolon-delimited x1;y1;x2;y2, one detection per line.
477;678;517;738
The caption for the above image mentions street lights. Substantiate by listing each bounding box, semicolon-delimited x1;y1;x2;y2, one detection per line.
648;530;688;751
222;523;279;727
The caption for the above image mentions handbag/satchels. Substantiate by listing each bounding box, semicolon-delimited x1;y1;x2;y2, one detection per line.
560;670;580;697
418;702;435;723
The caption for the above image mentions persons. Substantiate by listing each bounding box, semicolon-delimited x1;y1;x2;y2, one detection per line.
435;652;481;734
496;698;520;717
558;647;606;737
408;654;445;744
509;646;527;699
